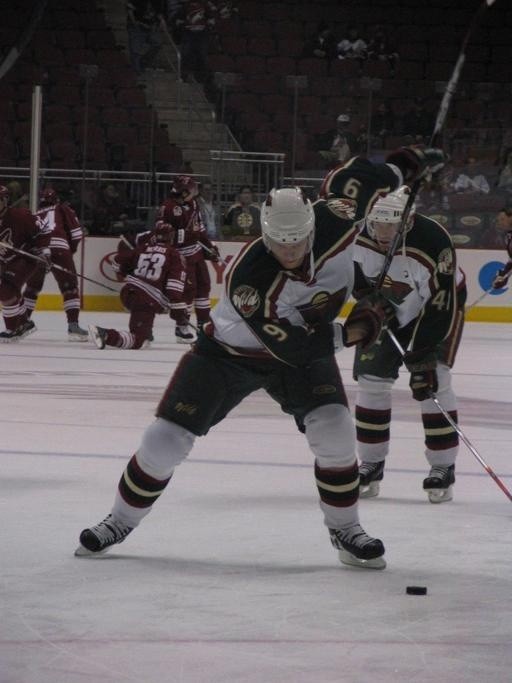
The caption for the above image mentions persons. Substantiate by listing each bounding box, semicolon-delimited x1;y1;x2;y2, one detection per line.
0;100;512;351
335;25;371;63
299;21;339;61
349;185;468;489
77;143;449;561
364;22;401;79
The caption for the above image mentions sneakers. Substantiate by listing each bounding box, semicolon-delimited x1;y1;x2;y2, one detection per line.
175;326;193;338
424;464;455;488
328;526;382;559
79;514;133;552
359;460;385;487
1;320;110;348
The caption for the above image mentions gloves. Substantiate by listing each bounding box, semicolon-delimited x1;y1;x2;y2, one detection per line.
111;262;129;274
409;371;438;401
35;248;52;271
204;245;220;263
345;294;385;351
388;146;451;183
170;304;187;320
492;270;507;288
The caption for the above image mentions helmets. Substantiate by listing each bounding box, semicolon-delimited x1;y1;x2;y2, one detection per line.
366;187;414;241
260;186;317;253
1;185;9;196
152;175;198;249
40;189;56;205
497;208;512;232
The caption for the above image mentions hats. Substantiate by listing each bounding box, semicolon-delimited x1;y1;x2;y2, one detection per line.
337;115;350;124
